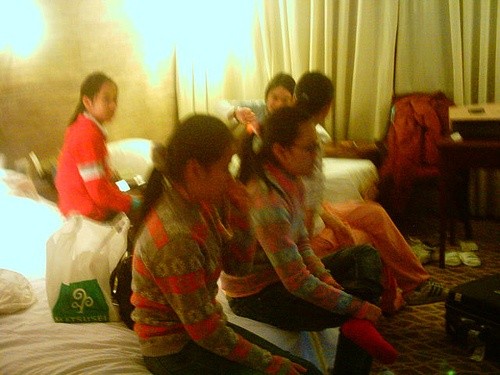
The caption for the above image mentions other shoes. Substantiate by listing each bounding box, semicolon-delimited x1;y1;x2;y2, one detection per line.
459;251;482;267
405;279;461;306
445;251;461;266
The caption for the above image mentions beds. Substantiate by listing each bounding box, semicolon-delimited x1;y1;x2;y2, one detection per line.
0;169;339;375
228;153;380;205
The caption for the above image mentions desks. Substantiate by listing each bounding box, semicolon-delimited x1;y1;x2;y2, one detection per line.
453;137;500;240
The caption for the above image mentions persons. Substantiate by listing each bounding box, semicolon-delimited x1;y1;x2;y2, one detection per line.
226;72;295;178
221;105;402;375
291;71;449;315
55;71;142;223
127;112;324;375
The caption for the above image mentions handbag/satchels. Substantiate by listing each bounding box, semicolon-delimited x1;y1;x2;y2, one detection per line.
44;211;130;324
109;255;136;332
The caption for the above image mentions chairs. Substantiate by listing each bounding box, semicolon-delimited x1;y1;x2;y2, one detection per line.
375;91;456;269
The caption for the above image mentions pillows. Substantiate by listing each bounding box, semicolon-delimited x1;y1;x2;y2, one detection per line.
106;138;154;179
